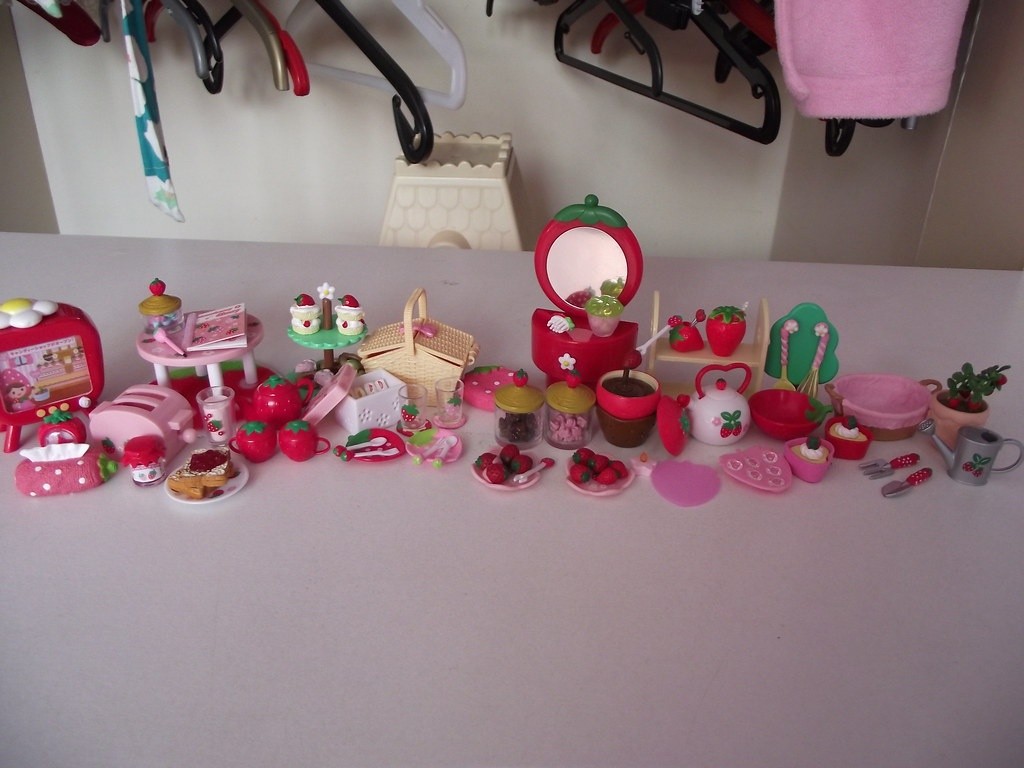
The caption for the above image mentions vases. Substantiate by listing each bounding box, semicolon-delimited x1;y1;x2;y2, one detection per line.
931;390;990;452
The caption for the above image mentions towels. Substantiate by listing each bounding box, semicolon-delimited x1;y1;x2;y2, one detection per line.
771;0;972;118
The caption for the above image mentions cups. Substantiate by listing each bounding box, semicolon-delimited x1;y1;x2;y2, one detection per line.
398;384;428;428
434;377;464;424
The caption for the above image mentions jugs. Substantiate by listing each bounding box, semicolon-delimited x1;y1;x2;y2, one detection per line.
234;375;314;421
685;363;753;446
918;417;1024;487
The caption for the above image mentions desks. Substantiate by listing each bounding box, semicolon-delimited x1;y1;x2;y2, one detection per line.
0;232;1024;768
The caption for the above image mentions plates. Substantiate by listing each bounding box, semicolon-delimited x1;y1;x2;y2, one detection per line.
163;459;249;505
565;450;635;497
470;448;541;490
406;428;462;465
345;427;404;462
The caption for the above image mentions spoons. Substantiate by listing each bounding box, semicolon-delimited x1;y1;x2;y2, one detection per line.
341;448;399;462
333;436;387;458
882;468;932;497
433;435;458;468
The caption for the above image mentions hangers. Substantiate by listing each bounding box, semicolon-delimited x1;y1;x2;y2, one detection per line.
487;0;920;156
18;0;469;163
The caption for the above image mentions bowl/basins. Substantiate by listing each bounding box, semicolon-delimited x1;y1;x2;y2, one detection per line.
750;389;832;438
823;372;943;441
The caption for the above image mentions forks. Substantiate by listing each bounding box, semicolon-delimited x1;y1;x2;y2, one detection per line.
412;436;446;464
858;452;920;479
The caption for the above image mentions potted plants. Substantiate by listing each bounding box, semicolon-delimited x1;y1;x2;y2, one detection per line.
597;350;661;448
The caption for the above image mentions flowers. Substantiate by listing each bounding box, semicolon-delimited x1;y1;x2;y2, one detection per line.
947;363;1011;409
